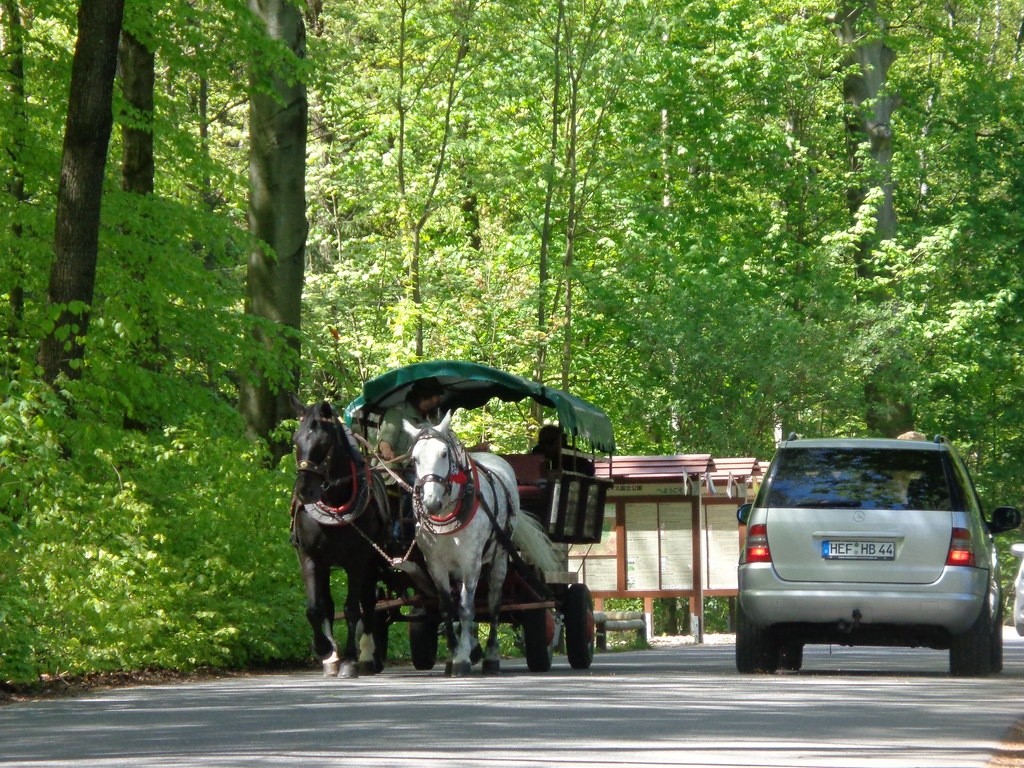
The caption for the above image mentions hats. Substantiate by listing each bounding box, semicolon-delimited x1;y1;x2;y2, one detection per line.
408;377;444;401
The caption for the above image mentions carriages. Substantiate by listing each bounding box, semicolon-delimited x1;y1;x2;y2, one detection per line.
287;361;615;674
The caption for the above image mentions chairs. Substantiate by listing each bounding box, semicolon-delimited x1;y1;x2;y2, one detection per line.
894;478;945;511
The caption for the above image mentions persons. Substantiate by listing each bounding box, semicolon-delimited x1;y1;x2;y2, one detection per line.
530;423;566;490
367;375;445;557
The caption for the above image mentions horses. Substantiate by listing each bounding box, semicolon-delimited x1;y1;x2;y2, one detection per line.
399;408;571;679
289;401;391;682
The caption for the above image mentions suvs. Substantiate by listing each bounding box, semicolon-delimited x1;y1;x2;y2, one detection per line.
734;430;1023;676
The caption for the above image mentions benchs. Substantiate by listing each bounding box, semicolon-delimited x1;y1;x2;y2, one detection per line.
494;453;546;497
544;471;614;541
